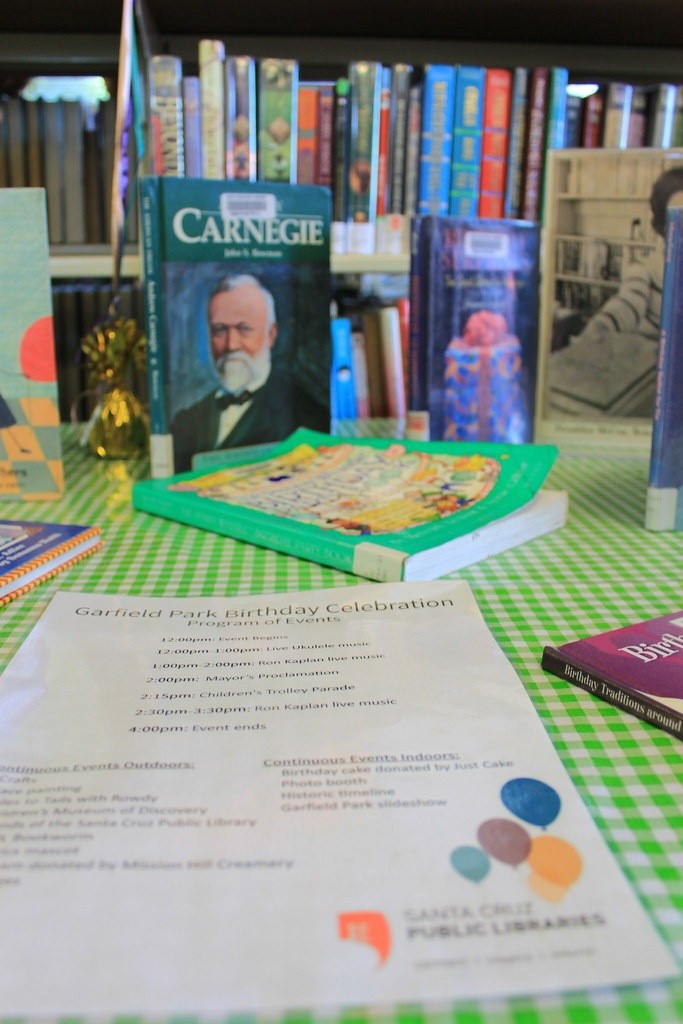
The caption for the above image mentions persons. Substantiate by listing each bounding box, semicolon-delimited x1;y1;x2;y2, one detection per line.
168;273;331;474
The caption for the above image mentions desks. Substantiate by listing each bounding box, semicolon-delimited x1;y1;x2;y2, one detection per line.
0;421;683;1024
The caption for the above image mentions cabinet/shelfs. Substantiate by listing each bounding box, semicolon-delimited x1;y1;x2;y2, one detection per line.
0;0;683;279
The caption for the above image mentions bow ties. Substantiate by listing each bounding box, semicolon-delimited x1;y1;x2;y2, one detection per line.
216;390;256;410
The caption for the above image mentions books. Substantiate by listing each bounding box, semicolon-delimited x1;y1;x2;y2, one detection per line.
0;519;104;607
0;38;682;482
541;609;683;740
643;208;683;533
134;427;571;583
